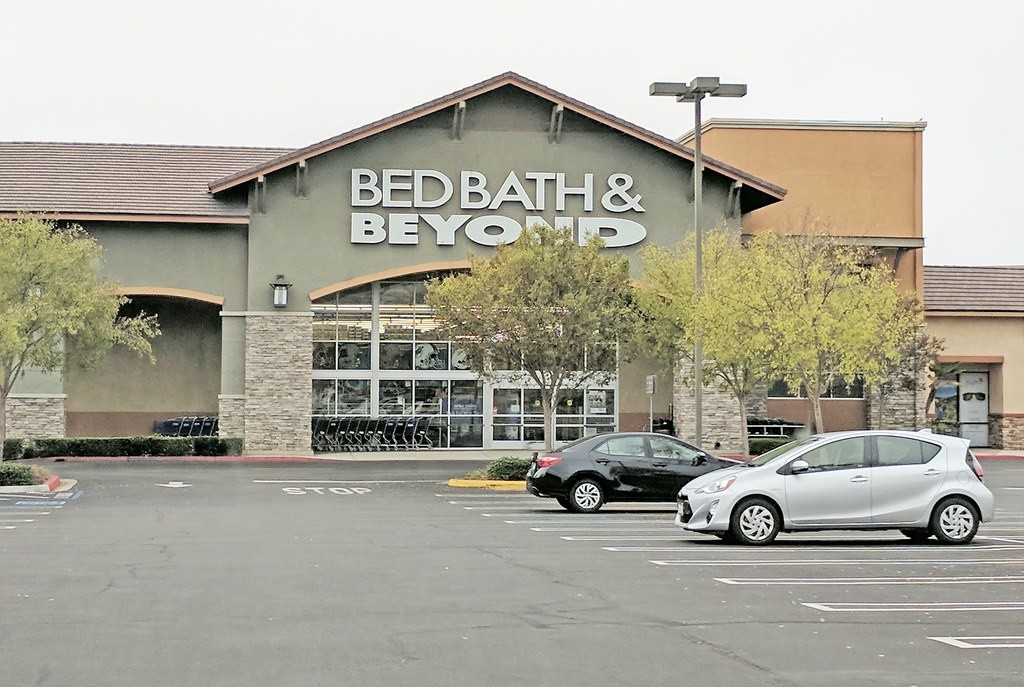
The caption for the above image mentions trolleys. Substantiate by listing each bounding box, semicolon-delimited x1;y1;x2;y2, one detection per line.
651;417;805;442
153;410;435;453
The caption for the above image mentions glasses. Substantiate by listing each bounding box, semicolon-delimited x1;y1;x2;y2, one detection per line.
964;393;985;401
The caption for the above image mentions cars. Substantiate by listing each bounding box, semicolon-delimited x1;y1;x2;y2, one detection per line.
672;428;995;549
524;428;745;515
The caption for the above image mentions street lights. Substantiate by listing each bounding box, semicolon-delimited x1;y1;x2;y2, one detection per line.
649;77;750;449
